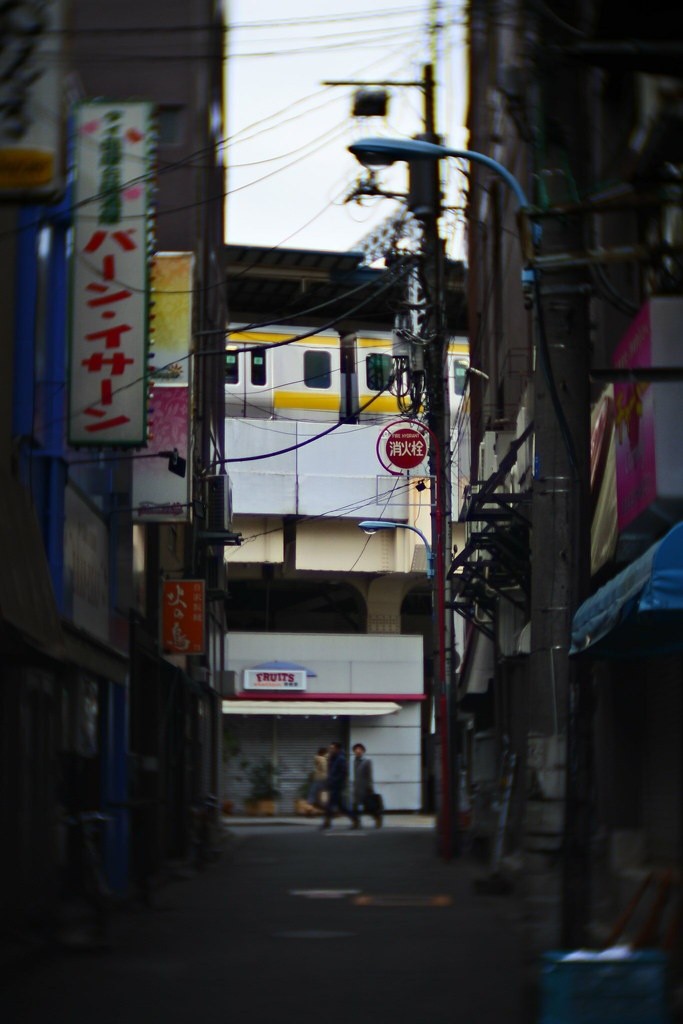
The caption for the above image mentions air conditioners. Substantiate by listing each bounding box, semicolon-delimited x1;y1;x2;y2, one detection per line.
207;473;233;533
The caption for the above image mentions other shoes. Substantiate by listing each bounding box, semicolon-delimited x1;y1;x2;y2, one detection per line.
351;820;361;829
375;817;382;827
322;821;331;829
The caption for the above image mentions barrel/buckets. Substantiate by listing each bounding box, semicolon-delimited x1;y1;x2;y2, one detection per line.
538;948;668;1024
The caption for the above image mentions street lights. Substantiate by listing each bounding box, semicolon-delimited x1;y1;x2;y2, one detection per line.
358;521;456;862
345;133;571;1024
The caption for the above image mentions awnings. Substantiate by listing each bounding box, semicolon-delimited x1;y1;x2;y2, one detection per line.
220;699;402;715
567;521;683;662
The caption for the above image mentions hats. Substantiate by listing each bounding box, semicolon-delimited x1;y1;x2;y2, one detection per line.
352;743;366;754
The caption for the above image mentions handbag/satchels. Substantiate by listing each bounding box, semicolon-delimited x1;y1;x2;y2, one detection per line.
364;794;383;813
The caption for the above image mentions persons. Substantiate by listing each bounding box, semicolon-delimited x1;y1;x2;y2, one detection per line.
320;742;365;831
309;748;330;810
350;743;382;832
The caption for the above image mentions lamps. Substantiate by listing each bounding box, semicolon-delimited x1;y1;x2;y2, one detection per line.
68;446;187;479
